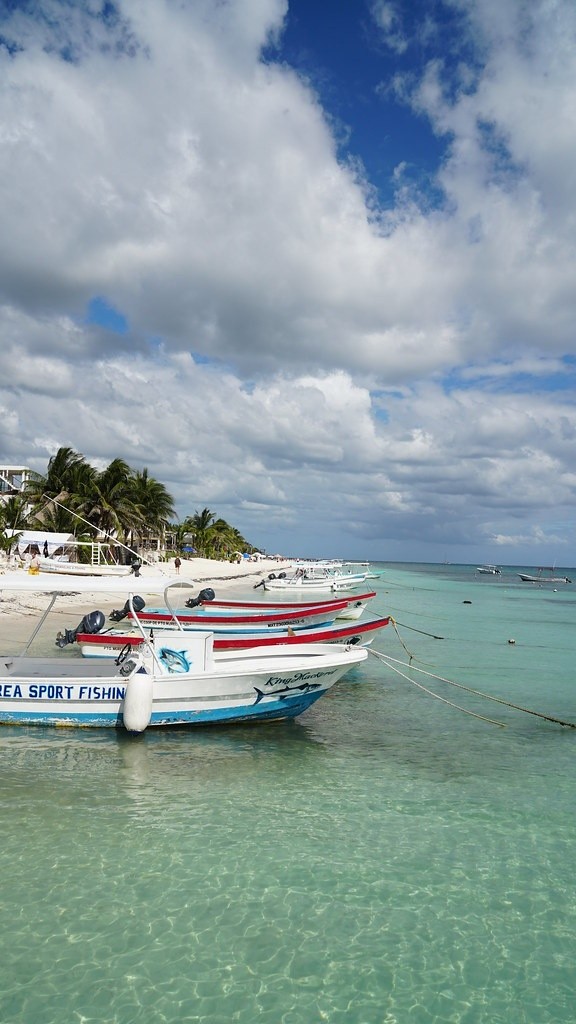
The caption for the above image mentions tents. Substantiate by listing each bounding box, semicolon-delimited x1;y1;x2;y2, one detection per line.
2;529;76;559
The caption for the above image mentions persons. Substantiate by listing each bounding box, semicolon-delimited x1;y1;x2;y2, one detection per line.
174;556;181;574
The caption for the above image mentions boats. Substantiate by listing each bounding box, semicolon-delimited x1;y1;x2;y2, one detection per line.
516;568;572;583
1;575;371;729
55;609;391;659
107;594;349;633
477;564;501;574
184;588;377;620
32;541;142;575
252;562;386;591
